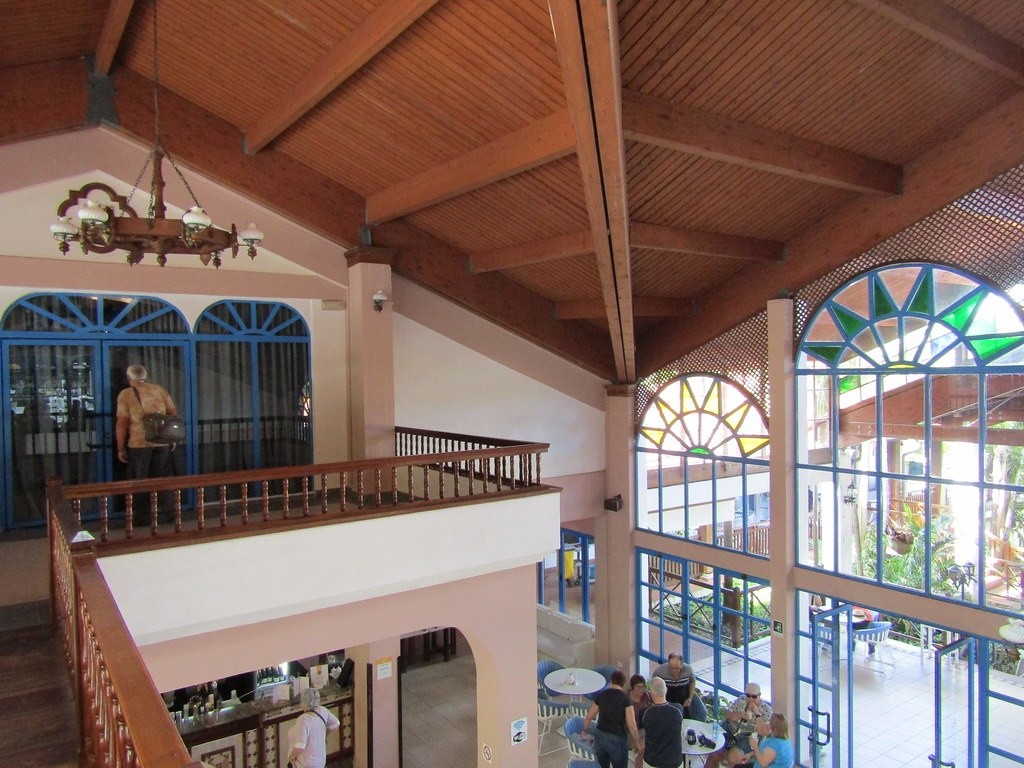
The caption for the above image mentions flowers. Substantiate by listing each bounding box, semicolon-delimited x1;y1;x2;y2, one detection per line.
891;531;915;544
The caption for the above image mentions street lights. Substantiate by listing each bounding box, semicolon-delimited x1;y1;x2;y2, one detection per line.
948;562;976;601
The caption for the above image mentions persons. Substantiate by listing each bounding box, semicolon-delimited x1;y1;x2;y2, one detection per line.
739;712;794;768
288;687;341;768
703;682;773;768
622;673;654;731
580;671;642;768
116;364;180;527
809;592;875;660
652;651;696;768
634;676;684;768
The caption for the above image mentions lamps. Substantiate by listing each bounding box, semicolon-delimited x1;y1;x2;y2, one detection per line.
51;0;264;270
844;481;856;504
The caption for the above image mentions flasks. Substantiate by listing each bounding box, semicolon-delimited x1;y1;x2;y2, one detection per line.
687;729;696;745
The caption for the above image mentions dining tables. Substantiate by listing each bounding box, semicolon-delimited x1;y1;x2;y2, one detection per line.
821;605;866;659
544;668;607;702
681;719;726;768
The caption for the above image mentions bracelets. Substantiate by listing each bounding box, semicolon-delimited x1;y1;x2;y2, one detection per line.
687;698;692;702
755;713;762;718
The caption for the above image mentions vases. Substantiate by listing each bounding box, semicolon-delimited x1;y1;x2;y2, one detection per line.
893;539;912;555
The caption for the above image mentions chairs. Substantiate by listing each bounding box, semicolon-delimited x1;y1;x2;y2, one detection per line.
564;716;599;762
538;698;569;756
856;621;892;659
580;664;619;703
568;755;602;768
537;660;566;700
810;624;832;651
572;703;599;724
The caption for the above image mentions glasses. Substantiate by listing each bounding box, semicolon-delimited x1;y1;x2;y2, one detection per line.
744;692;760;699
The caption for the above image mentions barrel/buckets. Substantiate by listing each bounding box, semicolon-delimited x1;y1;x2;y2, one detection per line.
588;564;595;580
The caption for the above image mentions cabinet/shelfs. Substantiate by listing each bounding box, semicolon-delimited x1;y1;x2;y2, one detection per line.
174;678;354;768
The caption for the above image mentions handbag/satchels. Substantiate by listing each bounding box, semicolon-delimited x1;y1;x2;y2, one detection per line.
142;413;186;445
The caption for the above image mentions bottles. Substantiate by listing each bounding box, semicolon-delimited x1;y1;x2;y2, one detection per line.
256;663;286;687
712;720;718;740
750;733;758;750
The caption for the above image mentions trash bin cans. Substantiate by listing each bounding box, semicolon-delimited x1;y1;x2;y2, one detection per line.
555;546;575;587
